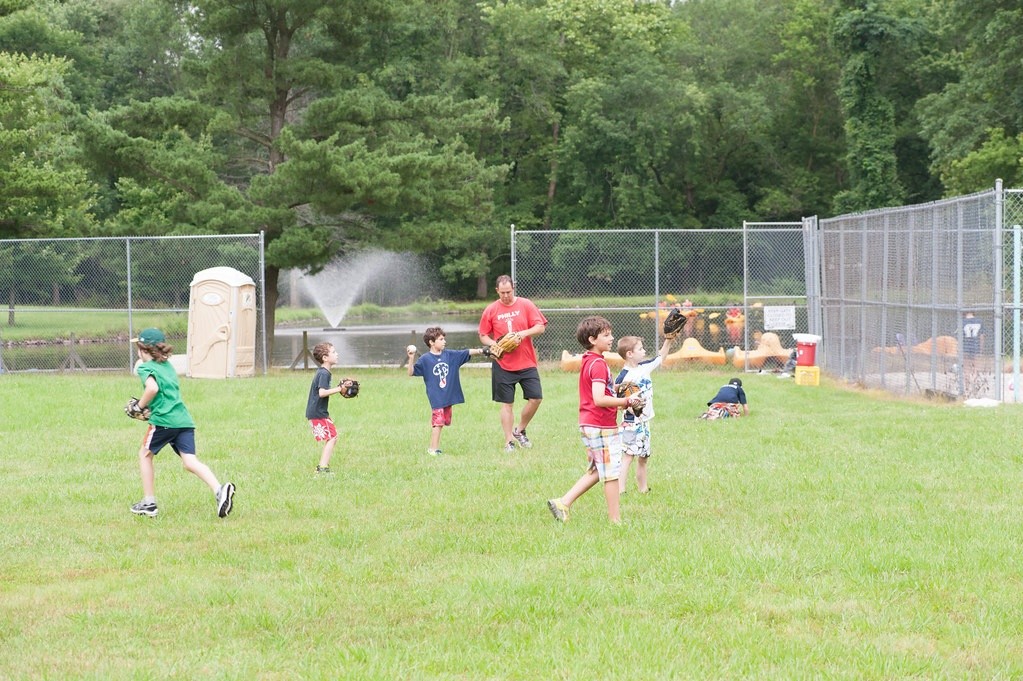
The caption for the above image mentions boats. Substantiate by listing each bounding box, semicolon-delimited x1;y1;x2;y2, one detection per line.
725;315;744;323
726;332;793;370
560;349;625;371
662;337;727;365
658;318;720;343
871;335;958;357
639;310;698;319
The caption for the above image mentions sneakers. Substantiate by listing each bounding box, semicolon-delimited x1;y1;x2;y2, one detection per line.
129;498;159;517
315;462;335;474
511;427;534;451
546;497;572;528
215;481;236;519
505;441;517;455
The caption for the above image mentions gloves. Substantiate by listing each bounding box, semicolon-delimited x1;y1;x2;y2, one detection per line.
625;393;647;410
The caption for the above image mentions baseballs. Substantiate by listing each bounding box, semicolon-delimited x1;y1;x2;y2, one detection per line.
407;344;416;353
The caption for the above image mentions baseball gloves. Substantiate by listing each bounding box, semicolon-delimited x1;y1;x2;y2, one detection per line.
496;331;522;353
124;396;150;421
617;381;645;417
481;345;504;361
662;308;689;340
338;379;360;398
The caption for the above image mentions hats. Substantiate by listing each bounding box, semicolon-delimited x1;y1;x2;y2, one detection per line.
129;328;167;346
728;378;743;386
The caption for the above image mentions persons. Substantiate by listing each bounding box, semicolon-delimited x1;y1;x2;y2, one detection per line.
124;327;237;519
406;327;491;459
614;309;687;498
305;341;348;476
698;378;750;420
954;303;984;398
548;316;647;529
478;275;548;455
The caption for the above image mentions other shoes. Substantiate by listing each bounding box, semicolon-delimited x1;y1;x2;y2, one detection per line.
427;447;444;457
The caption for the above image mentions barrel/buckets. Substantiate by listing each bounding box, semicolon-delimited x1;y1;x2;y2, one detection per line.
797;342;816;366
